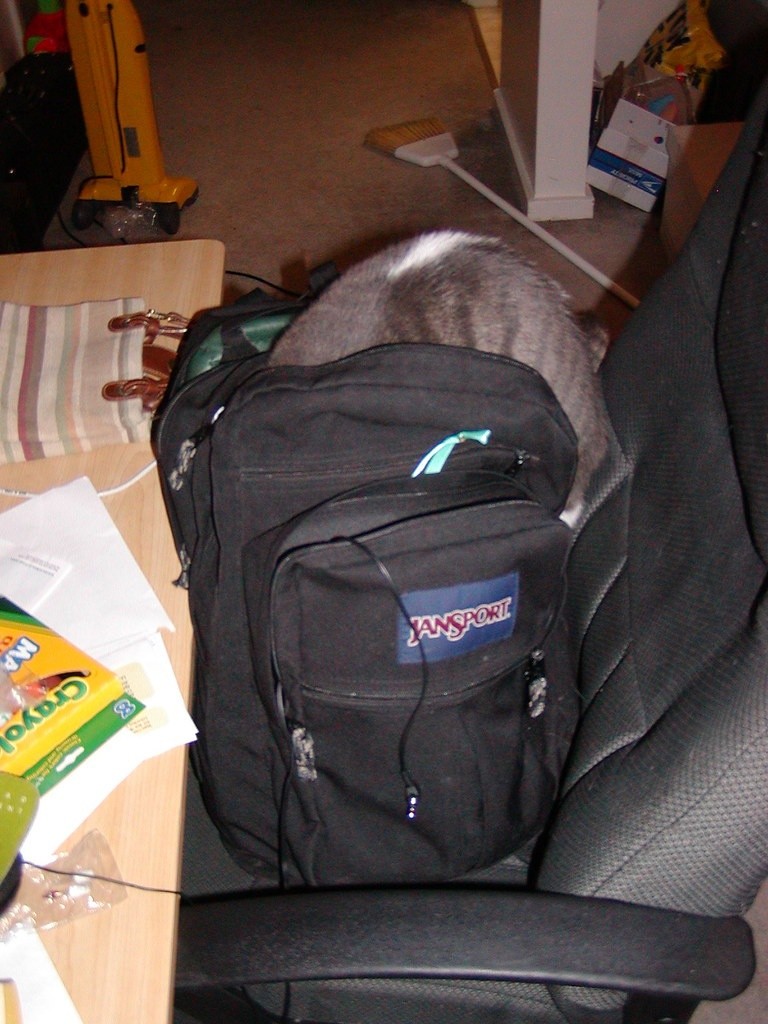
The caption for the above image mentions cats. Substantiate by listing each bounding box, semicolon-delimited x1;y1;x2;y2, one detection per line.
267;227;609;530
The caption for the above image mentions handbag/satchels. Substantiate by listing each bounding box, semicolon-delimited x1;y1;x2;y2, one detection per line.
0;298;191;465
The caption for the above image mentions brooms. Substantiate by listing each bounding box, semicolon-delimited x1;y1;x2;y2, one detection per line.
361;110;644;310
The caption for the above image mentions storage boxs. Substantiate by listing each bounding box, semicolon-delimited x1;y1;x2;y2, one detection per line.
0;594;146;797
585;61;680;212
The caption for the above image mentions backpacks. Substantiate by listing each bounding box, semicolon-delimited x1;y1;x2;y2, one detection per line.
150;290;581;888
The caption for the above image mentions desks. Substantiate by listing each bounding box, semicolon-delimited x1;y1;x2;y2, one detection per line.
1;238;226;1024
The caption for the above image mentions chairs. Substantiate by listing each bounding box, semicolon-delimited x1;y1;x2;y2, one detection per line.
174;91;768;1024
